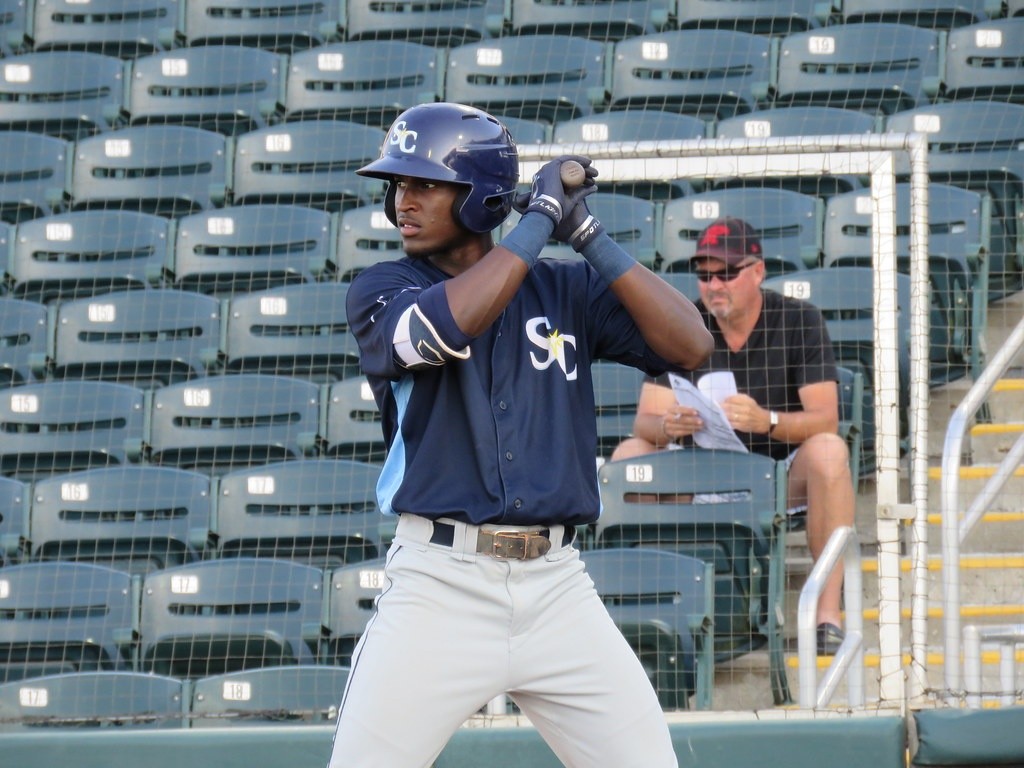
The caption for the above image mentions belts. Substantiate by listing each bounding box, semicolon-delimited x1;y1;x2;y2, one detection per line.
430;521;576;560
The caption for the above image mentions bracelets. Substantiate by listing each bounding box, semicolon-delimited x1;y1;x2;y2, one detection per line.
499;212;554;265
661;419;673;441
766;411;778;435
581;231;635;285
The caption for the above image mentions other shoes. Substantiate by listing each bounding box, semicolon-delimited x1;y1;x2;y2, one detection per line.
817;624;846;656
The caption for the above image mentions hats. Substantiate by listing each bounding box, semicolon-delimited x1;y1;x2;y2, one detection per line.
691;217;763;267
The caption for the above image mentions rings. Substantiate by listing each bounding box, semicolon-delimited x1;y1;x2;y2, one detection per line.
676;414;680;421
735;414;737;421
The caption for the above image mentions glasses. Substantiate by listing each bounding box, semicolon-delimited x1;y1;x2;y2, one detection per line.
695;261;758;283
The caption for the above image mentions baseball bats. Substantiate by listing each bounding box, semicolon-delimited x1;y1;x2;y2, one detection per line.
560;160;586;188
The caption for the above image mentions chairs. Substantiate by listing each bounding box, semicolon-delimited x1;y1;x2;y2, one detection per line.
0;0;1024;736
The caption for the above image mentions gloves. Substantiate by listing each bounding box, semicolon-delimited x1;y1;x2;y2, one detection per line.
516;190;604;254
523;154;600;229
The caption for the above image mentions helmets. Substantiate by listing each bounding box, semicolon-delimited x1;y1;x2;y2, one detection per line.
355;103;520;235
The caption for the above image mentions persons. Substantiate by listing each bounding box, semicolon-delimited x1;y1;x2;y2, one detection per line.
326;103;715;768
611;219;854;656
